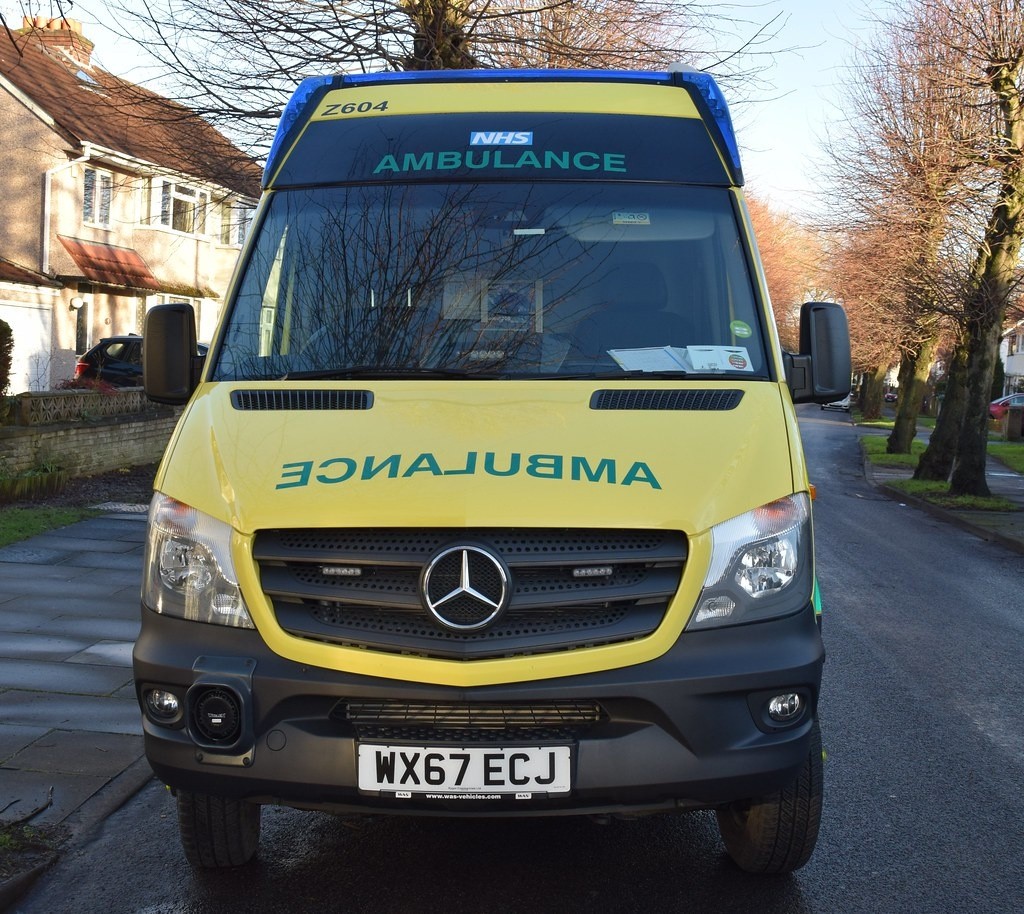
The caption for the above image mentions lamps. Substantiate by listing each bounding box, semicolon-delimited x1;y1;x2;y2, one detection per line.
69;296;84;311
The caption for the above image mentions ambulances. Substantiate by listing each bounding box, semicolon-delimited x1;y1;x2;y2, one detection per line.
132;69;850;879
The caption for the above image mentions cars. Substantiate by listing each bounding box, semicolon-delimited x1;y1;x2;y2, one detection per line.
821;392;854;413
989;393;1024;420
74;333;210;389
884;388;899;402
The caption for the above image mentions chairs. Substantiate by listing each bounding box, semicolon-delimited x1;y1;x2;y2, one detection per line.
337;243;445;355
573;260;695;364
